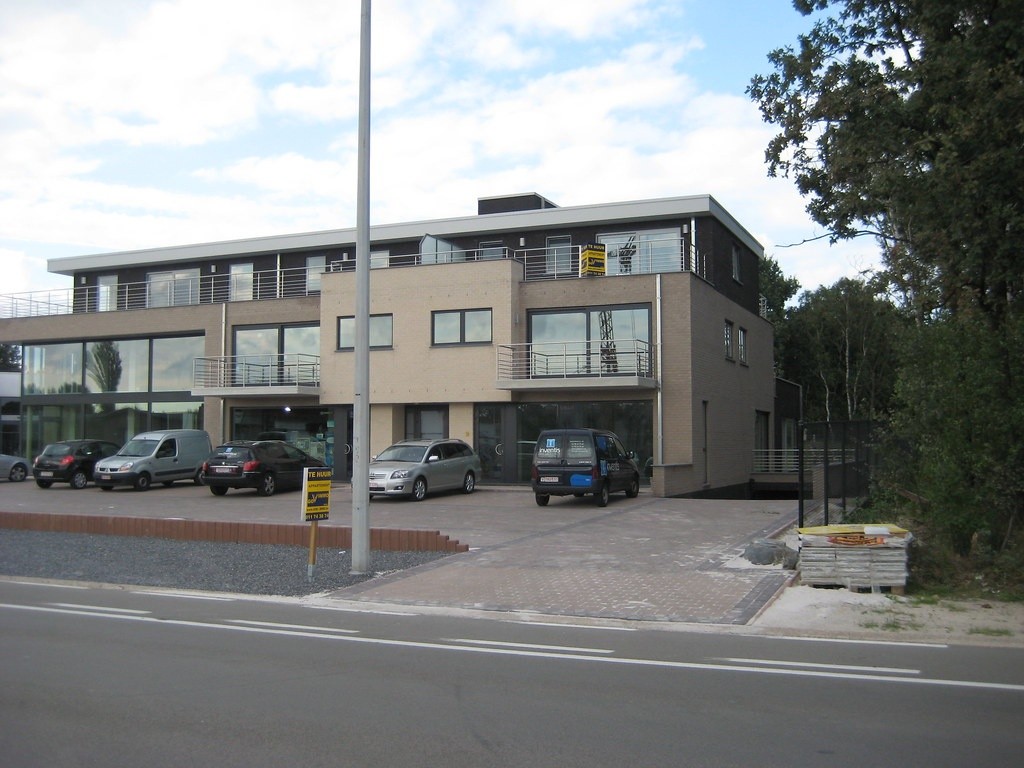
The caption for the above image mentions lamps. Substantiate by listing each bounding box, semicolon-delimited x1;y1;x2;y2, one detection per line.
210;264;217;273
342;253;349;261
683;224;689;234
520;237;525;247
80;275;86;285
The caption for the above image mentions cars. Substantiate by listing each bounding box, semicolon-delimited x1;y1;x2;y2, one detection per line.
199;437;325;497
492;438;538;481
0;453;34;483
32;437;138;492
349;437;483;501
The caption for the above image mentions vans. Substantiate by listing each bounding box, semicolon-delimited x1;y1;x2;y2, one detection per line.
530;426;641;508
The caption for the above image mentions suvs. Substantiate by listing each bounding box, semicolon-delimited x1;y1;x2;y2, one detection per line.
92;428;214;491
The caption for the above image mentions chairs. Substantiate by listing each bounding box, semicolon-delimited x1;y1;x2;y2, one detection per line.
400;448;418;461
